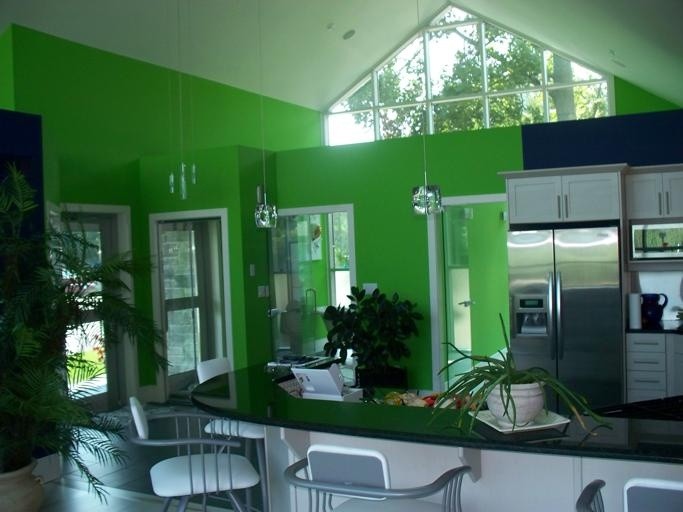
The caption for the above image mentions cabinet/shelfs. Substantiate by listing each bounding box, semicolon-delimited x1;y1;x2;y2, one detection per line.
626;162;683;220
496;163;626;224
624;329;682;404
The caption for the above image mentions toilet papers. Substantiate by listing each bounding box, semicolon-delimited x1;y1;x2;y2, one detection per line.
628;290;643;330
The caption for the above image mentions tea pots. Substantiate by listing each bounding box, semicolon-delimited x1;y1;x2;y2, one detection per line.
639;293;667;326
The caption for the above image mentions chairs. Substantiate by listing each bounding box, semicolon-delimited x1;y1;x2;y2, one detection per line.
575;476;683;512
194;358;268;511
282;441;469;512
116;383;268;512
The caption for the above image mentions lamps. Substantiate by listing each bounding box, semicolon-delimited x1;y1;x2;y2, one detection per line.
252;2;279;230
407;0;445;214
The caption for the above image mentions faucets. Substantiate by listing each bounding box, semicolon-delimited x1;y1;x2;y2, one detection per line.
305;288;316;313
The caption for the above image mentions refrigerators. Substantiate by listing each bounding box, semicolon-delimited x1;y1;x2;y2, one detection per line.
505;227;622;417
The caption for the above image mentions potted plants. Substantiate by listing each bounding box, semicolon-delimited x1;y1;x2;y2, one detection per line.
419;314;603;439
1;163;171;511
